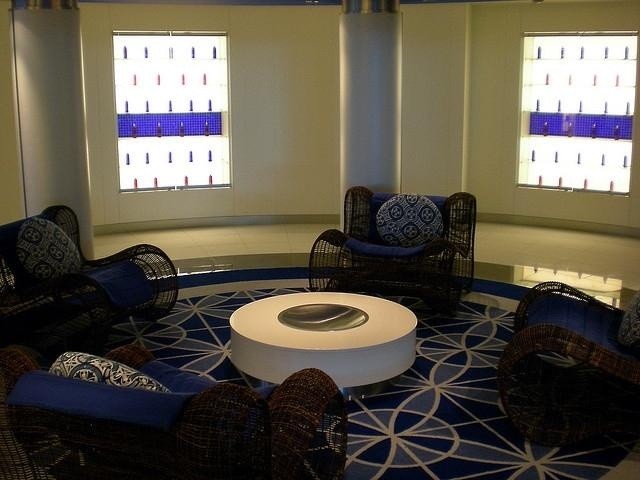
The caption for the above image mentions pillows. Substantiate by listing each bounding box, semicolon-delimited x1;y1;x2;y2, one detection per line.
376;192;443;248
618;289;640;352
17;217;82;282
49;351;175;397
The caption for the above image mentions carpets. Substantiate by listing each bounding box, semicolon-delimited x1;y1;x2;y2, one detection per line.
0;266;640;480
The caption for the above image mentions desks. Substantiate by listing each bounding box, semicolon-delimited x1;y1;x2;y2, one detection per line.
228;291;418;398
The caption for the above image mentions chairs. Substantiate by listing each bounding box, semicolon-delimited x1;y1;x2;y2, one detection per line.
1;338;349;480
497;280;639;446
1;205;178;356
309;185;478;316
